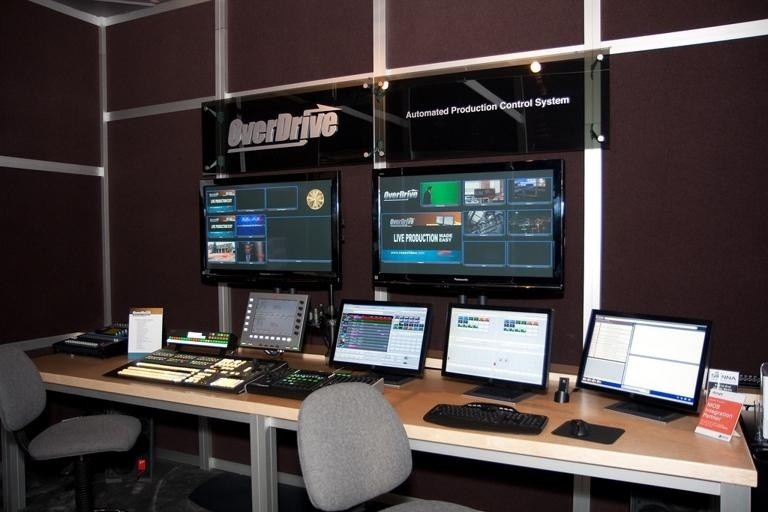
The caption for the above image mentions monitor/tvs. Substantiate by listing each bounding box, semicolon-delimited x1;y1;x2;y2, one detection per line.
441;302;555;402
328;298;433;385
576;309;712;423
371;158;566;299
240;290;309;354
199;169;342;291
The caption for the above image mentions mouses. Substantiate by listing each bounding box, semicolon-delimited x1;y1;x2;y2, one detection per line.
572;419;589;437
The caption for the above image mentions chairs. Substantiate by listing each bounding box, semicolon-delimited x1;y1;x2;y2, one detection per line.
0;341;149;511
287;377;485;512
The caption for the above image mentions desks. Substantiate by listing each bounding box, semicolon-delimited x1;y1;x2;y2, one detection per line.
27;335;760;511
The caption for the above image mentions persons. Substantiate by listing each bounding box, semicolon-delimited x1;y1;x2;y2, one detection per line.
241;244;258;263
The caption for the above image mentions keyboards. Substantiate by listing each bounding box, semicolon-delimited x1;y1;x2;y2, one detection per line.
423;402;549;435
